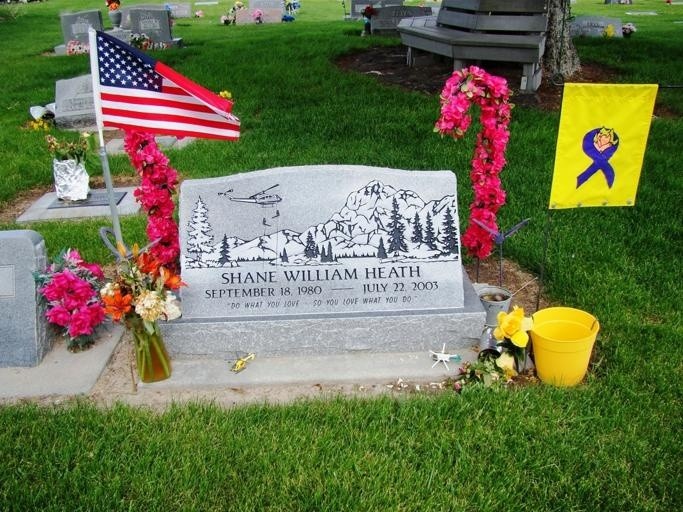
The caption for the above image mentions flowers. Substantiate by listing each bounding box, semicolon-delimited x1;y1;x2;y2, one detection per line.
97;241;188;383
30;109;90;160
453;307;532;391
435;66;514;291
128;33;149;46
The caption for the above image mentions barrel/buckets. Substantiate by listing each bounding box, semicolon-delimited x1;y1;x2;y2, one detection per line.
529;306;603;388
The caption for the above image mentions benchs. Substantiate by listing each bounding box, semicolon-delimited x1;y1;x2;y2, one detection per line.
396;0;552;92
370;5;433;39
349;0;403;21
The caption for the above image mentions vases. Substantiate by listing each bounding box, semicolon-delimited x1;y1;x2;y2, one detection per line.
53;162;88;201
122;316;170;383
140;42;147;51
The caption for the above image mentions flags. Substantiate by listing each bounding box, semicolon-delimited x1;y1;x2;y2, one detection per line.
548;82;659;209
89;29;240;140
